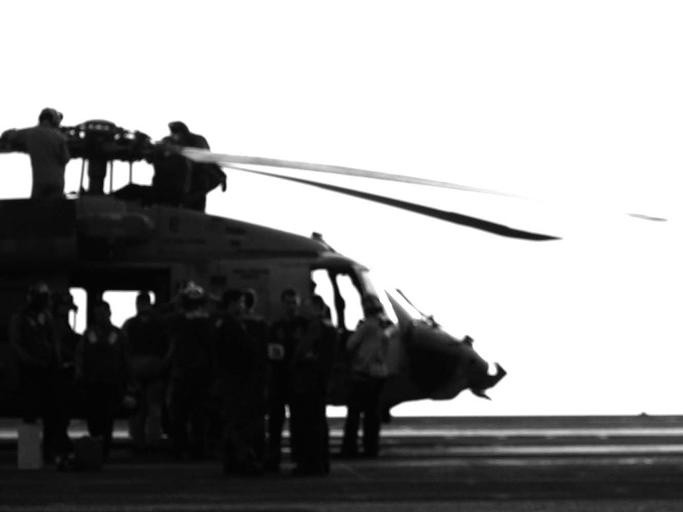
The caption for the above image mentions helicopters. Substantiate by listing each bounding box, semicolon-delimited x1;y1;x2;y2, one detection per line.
0;120;666;472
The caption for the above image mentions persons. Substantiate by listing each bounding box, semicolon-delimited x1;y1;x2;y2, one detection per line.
27;109;226;211
